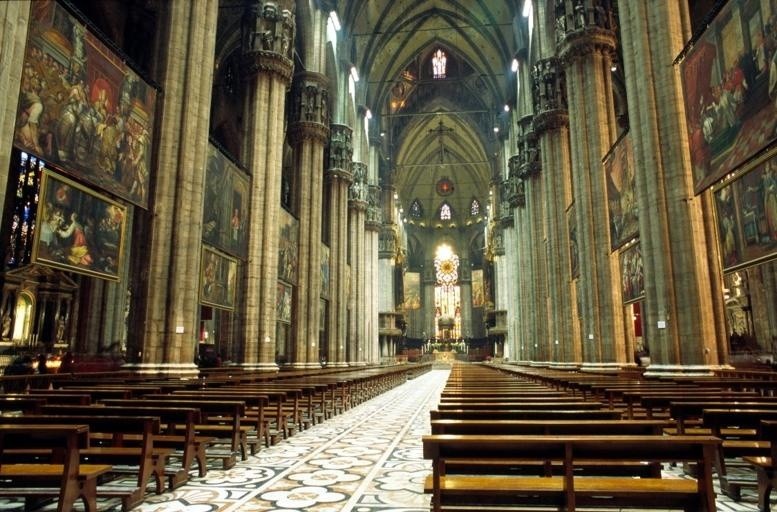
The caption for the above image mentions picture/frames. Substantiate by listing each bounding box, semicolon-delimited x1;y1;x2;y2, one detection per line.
12;0;162;213
197;244;238;311
678;0;776;196
30;167;128;283
275;279;293;326
201;135;252;263
566;199;581;281
709;148;777;274
319;298;326;331
319;240;331;302
618;240;646;305
604;134;638;254
721;263;776;355
278;201;299;288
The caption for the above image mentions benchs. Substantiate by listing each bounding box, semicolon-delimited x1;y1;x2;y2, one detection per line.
0;363;777;512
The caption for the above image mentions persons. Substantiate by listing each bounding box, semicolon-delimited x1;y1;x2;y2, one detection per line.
203;202;218;244
214;352;221;367
714;156;775;267
3;351;75;376
205;251;229;304
632;341;641;365
57;315;66;343
616;246;646;302
277;237;297;282
276;285;291;320
233;208;240;249
38;204;118;273
615;144;637;237
473;275;482;306
321;249;329;295
605;196;622;242
688;11;775;190
22;41;147;203
2;309;13;340
222;355;232;366
638;344;651;367
